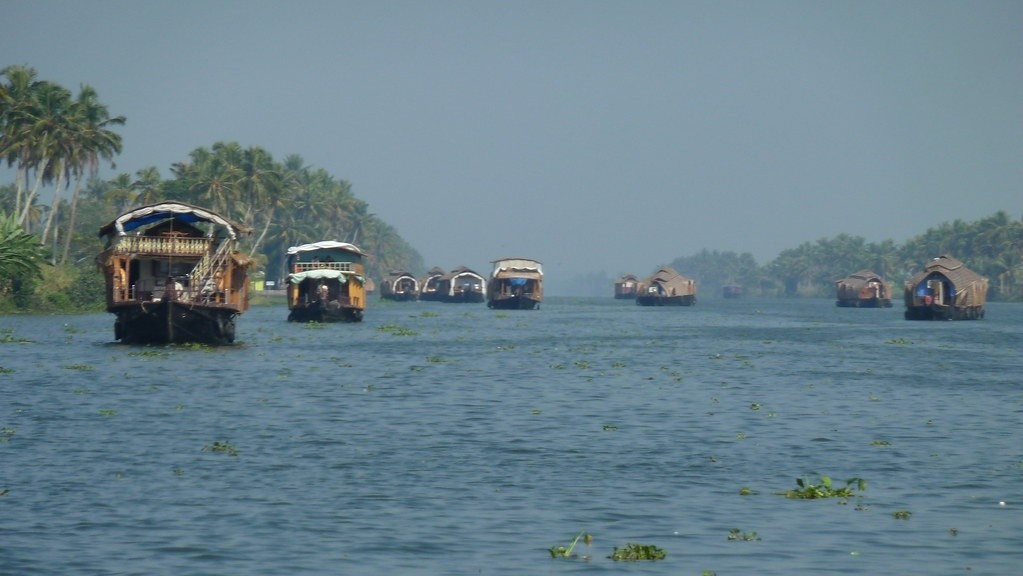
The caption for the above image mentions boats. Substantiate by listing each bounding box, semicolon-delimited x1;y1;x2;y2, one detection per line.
835;270;895;308
904;254;989;321
284;241;375;323
722;276;745;299
613;272;645;300
381;270;418;302
636;267;696;307
440;266;488;304
486;258;544;310
96;200;258;345
416;265;450;302
